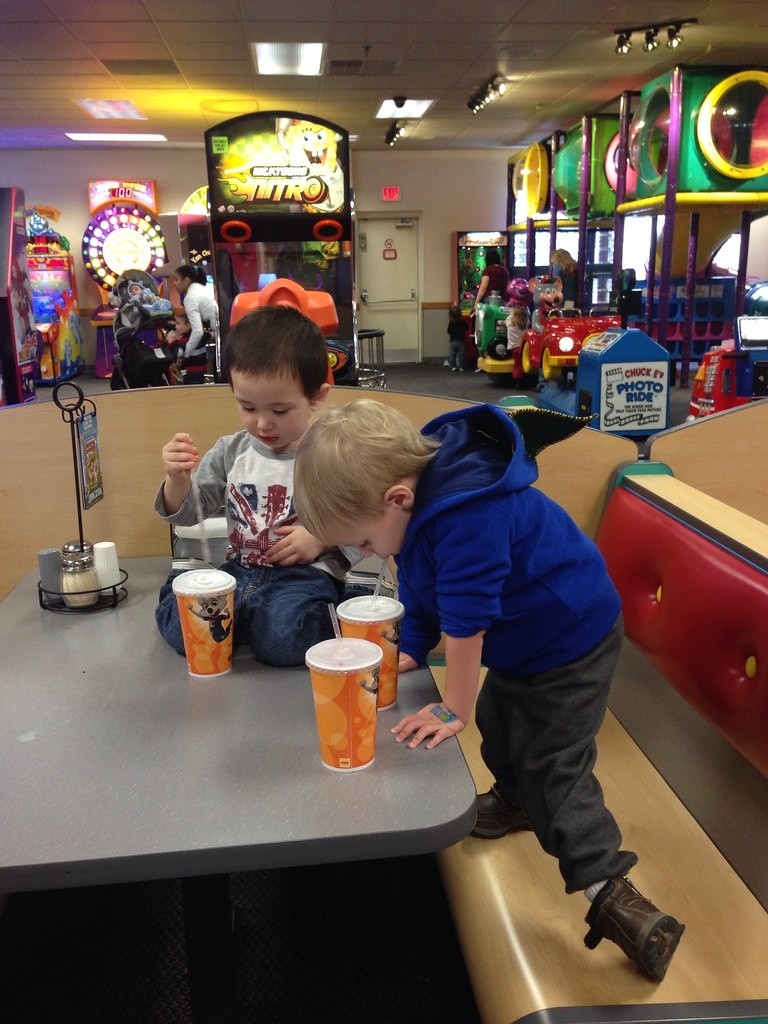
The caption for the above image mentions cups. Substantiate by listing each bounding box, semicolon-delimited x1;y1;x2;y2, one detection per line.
172;569;236;679
337;595;405;712
92;542;122;592
305;638;384;773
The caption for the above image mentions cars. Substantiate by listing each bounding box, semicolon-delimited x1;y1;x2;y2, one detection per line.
475;303;516;359
520;307;622;382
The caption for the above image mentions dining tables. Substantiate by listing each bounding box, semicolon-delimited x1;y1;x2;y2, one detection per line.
0;553;481;1024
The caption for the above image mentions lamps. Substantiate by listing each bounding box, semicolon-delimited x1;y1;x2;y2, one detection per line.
393;95;407;108
468;71;510;117
382;120;406;147
614;17;701;57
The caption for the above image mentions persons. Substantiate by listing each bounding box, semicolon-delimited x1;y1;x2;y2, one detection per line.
473;248;509;373
170;312;210;385
216;247;243;316
506;309;528;390
293;398;685;984
274;246;324;290
446;305;470;371
551;249;577;308
155;305;397;665
173;266;221;384
128;280;171;311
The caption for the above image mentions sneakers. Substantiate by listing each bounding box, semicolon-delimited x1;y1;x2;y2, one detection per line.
583;875;686;983
345;570;397;598
171;557;217;570
460;368;464;371
452;367;457;371
468;782;533;840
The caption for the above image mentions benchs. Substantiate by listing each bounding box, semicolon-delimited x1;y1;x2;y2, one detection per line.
645;399;768;529
426;461;768;1024
0;383;641;605
